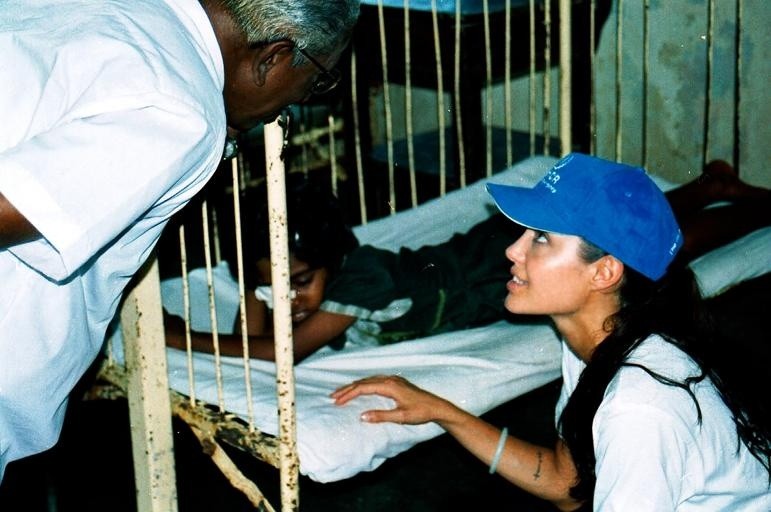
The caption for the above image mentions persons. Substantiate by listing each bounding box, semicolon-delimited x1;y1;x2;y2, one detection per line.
328;150;770;512
159;157;771;369
0;0;361;511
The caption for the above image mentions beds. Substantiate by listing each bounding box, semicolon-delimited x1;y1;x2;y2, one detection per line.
82;154;771;512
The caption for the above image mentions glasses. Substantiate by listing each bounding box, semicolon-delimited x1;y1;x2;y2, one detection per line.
296;44;343;94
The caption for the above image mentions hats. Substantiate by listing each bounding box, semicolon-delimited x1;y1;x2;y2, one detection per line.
483;150;683;280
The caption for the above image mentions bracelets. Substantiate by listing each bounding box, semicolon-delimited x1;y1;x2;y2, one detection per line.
488;426;509;476
